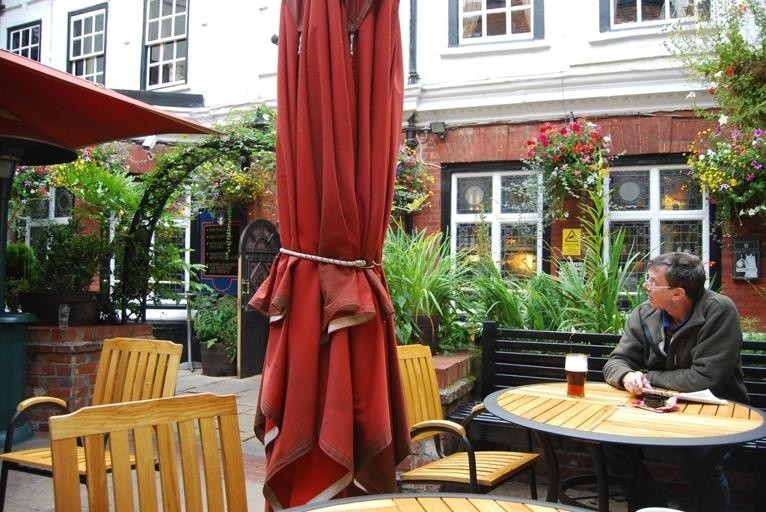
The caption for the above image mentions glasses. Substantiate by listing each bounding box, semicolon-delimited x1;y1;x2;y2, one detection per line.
642;271;672;291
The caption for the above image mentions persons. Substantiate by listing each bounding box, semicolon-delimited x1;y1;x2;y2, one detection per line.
602;252;751;511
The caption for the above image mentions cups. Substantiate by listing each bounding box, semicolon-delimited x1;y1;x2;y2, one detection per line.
58;303;71;327
565;354;589;397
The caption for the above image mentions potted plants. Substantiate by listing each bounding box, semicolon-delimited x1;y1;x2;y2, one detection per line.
194;290;237;377
19;215;117;326
380;210;479;357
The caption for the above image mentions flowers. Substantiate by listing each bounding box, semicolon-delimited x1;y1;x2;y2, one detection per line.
678;130;765;216
519;114;628;202
390;138;436;217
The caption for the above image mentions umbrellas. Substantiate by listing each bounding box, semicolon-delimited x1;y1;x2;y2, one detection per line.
247;0;412;512
0;46;230;149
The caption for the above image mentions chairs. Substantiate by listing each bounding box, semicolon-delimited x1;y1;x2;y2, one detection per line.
0;336;183;511
50;393;249;512
395;342;540;499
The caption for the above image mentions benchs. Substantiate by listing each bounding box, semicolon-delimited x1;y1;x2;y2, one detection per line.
451;322;765;465
